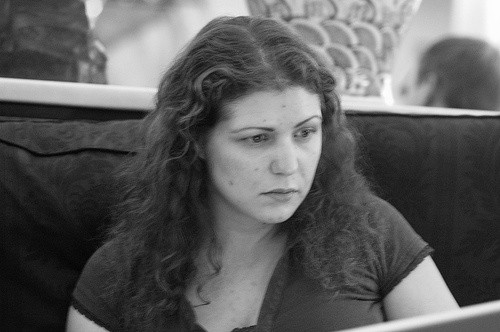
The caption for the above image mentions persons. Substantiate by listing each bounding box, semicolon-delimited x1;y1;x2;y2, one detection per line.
399;35;500;113
64;15;463;332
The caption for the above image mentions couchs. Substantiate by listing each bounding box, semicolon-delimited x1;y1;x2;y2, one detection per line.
1;111;500;332
